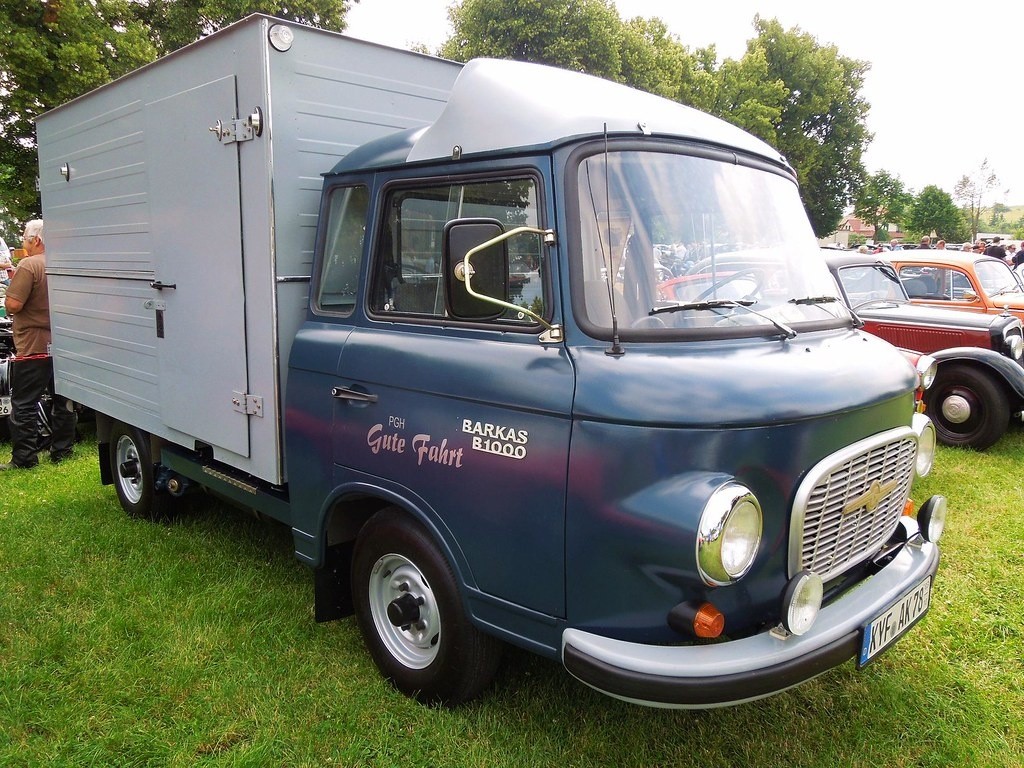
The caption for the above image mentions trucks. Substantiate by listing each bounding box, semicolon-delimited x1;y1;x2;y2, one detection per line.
32;12;948;712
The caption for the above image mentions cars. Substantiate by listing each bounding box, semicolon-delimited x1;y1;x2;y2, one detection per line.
652;250;770;311
689;252;1024;453
840;249;1024;324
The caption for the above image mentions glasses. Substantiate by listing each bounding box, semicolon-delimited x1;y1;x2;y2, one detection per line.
19;236;35;241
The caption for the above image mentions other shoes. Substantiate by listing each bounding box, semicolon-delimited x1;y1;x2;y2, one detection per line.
0;461;25;472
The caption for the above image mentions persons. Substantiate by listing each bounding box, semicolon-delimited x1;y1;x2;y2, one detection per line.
850;235;1024;294
0;218;76;472
0;235;12;286
